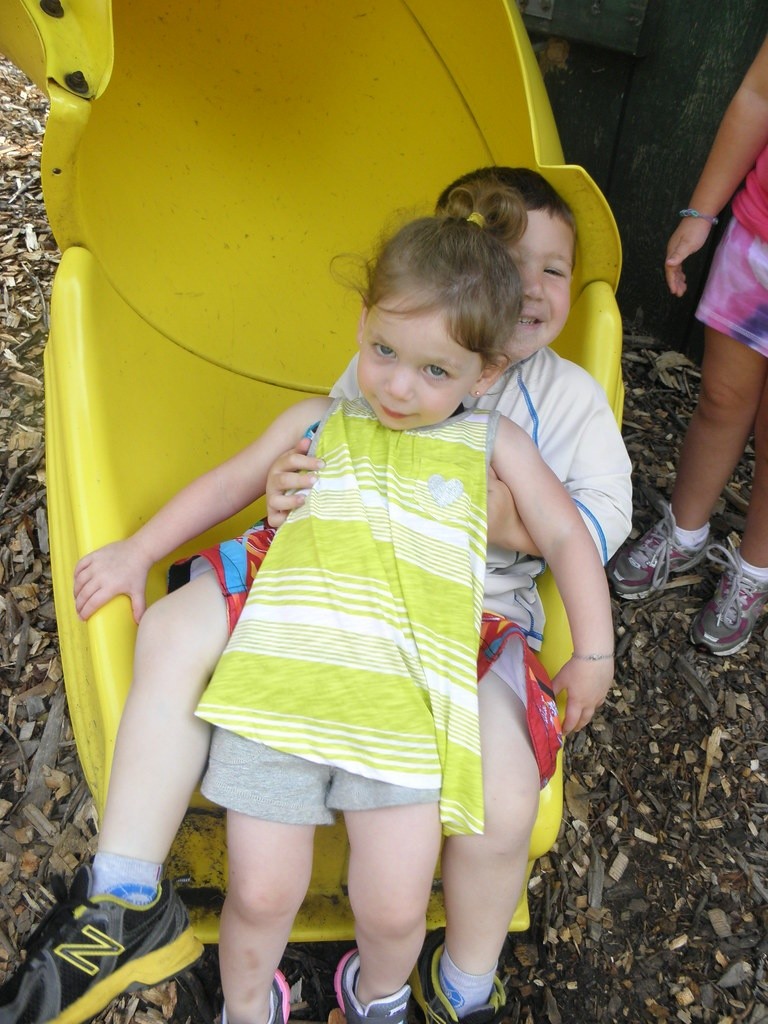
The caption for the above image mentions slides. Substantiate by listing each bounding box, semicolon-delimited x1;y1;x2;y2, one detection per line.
1;1;628;948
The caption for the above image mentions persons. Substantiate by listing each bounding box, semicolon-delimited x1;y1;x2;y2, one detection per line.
0;163;637;1024
608;23;768;654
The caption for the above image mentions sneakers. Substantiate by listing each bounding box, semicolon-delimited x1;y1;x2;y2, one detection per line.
222;968;291;1024
407;928;506;1024
609;501;710;600
688;536;768;656
0;864;204;1024
332;949;411;1024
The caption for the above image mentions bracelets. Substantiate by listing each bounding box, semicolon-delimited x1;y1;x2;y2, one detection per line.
572;650;614;662
678;208;719;225
